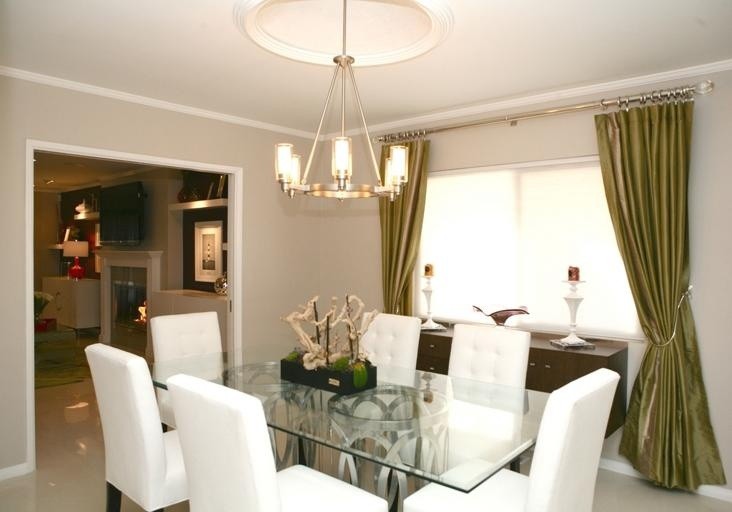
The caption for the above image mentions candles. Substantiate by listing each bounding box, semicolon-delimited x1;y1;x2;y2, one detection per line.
568;266;579;281
424;263;433;277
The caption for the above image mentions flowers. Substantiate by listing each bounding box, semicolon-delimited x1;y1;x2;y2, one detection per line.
34;292;55;322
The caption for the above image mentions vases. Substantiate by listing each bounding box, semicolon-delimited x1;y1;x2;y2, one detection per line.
34;319;56;333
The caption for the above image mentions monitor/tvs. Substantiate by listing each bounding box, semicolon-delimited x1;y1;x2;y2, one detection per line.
99;180;145;247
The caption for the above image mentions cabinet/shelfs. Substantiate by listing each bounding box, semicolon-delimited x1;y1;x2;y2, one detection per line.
151;289;227;361
416;319;628;438
42;278;101;336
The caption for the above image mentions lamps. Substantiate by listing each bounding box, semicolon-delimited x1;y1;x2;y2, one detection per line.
63;240;89;278
274;0;410;203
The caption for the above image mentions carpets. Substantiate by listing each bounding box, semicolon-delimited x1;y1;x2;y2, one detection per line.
35;325;101;389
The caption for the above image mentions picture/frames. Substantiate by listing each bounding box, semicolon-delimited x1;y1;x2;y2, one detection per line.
183;211;228;292
